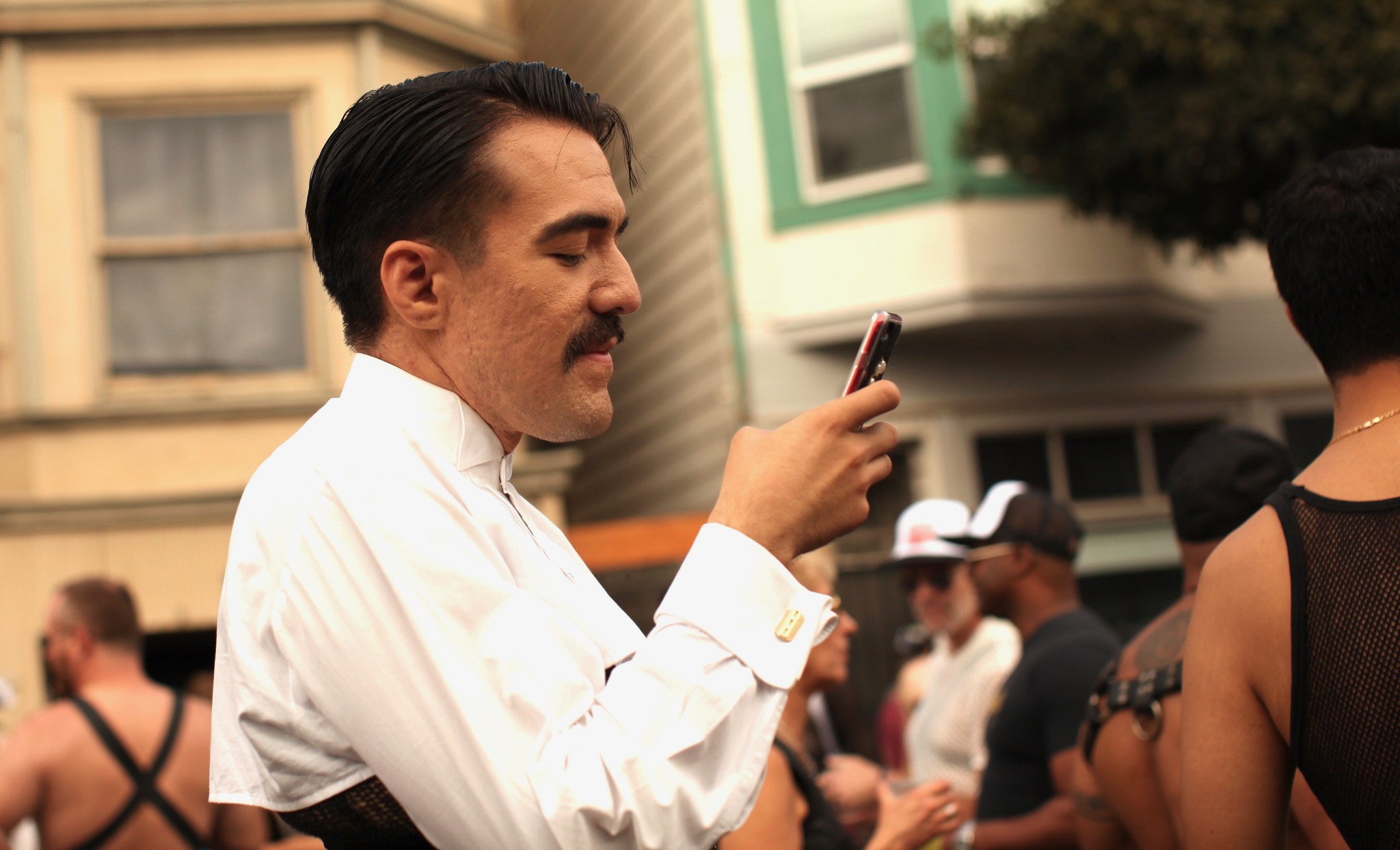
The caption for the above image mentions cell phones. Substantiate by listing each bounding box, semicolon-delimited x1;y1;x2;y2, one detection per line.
842;310;906;398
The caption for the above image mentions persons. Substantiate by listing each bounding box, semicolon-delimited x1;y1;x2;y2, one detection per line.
874;616;934;772
1077;425;1348;850
935;481;1124;850
204;62;903;850
1176;147;1400;850
707;545;960;850
813;496;1025;850
0;576;271;850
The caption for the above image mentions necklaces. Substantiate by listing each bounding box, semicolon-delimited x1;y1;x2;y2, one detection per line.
1322;409;1400;447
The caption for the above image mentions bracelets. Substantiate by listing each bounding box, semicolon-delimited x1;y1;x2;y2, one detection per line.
955;821;977;850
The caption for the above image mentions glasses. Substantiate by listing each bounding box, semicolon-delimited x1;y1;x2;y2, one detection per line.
899;560;962;595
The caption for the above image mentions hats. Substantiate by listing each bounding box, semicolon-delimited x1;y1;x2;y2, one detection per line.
938;480;1082;561
1167;427;1293;542
889;497;976;563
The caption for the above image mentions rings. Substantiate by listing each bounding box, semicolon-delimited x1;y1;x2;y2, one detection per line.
943;803;956;817
